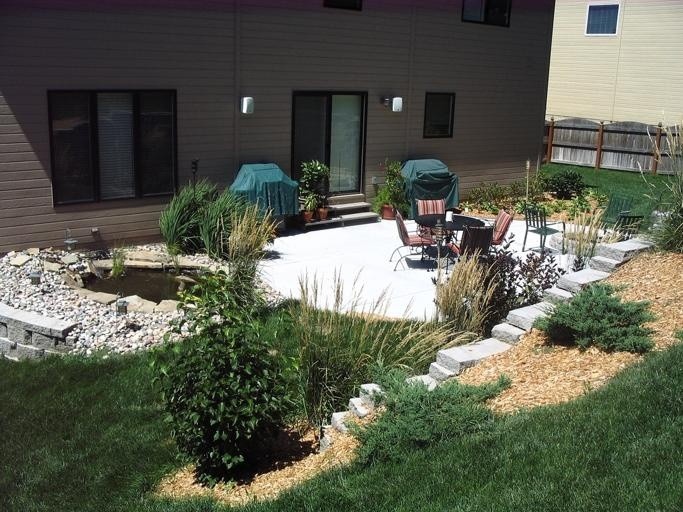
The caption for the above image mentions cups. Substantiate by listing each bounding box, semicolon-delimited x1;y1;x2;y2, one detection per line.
445;211;452;221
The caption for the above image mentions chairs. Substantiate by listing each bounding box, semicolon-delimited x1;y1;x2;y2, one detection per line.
388;192;645;275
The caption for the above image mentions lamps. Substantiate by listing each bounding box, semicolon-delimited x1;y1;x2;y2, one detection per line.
239;95;255;121
382;95;404;113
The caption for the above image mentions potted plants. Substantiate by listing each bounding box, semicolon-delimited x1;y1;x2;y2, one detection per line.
370;153;413;221
295;158;329;223
